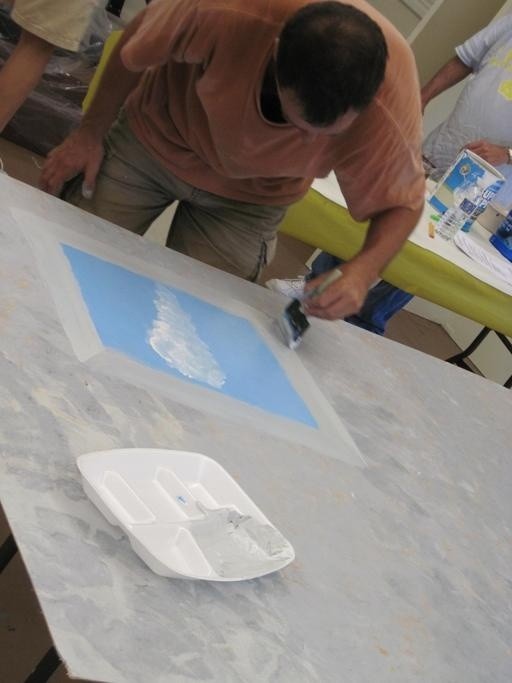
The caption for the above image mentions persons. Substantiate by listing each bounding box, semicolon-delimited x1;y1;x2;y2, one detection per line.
38;0;427;321
418;0;512;227
1;0;100;143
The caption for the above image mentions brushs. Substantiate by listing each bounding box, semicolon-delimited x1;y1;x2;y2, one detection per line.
271;269;344;350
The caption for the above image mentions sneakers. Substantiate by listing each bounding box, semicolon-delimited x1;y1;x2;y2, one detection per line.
265;275;306;297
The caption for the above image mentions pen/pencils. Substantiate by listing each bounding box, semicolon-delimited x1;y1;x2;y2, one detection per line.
429;213;444;239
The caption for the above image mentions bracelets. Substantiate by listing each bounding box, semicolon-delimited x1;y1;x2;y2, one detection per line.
505;143;512;166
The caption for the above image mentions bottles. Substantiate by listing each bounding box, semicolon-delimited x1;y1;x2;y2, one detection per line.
420;154;438;180
434;185;484;241
